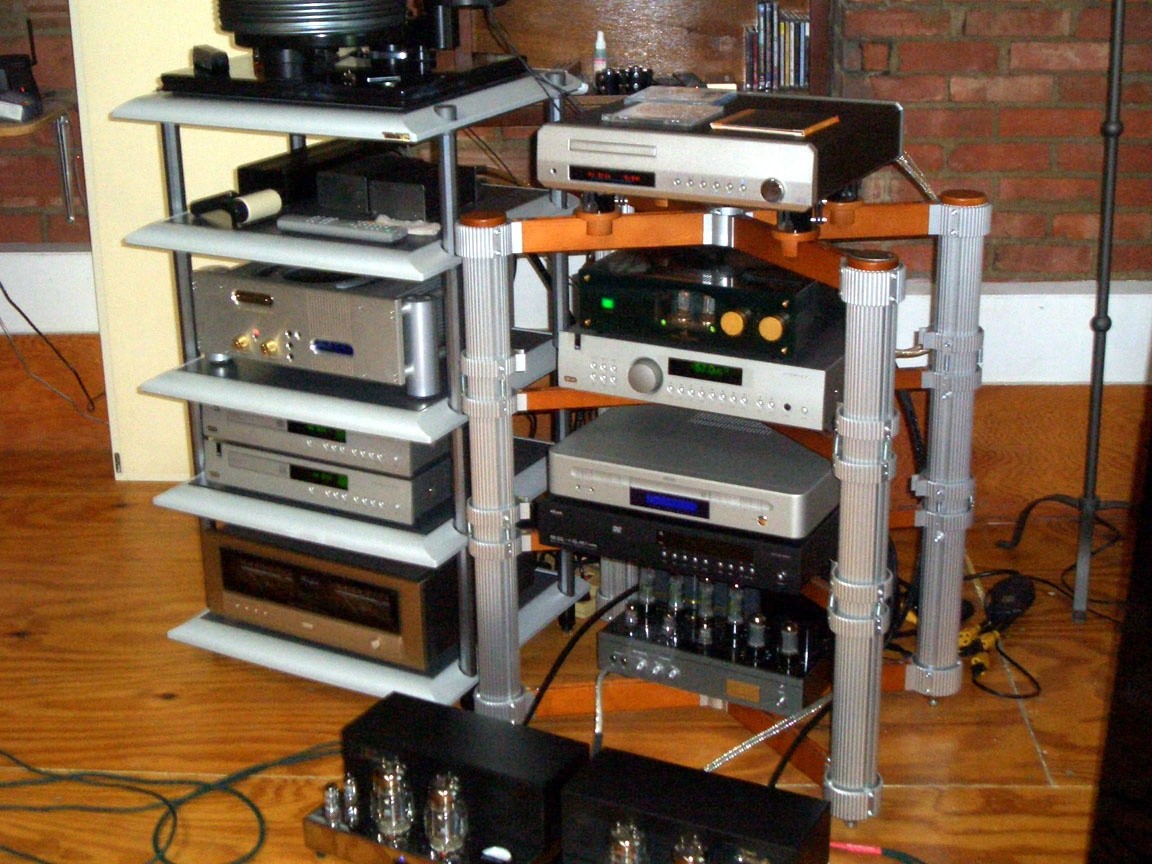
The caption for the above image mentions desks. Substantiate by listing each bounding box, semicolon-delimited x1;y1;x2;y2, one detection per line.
0;90;78;223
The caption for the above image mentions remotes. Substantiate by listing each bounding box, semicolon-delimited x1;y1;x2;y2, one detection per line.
276;213;410;242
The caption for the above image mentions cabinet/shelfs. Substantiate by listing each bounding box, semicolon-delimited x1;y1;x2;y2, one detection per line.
112;69;994;834
